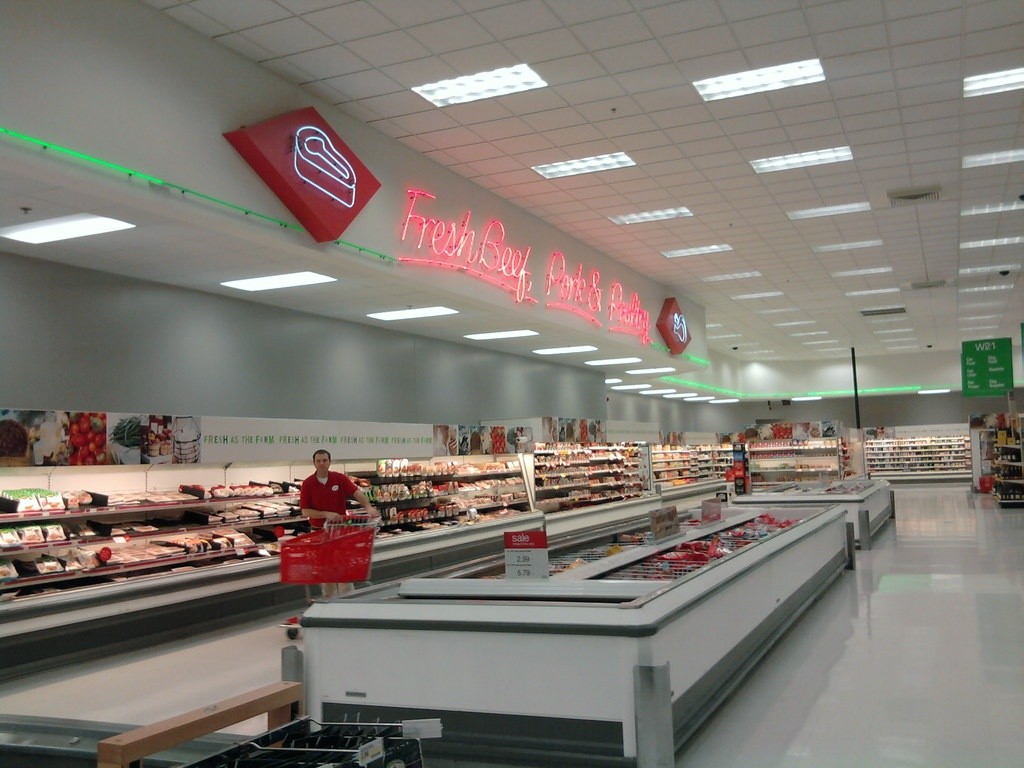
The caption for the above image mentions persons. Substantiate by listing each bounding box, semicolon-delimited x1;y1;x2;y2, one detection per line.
300;450;378;598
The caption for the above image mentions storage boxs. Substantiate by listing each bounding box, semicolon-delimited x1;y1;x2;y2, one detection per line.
997;431;1016;445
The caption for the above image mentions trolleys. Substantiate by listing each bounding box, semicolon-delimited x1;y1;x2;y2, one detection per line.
278;514;381;637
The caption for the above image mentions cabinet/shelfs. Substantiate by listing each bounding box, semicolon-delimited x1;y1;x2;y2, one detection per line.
866;437;967;471
992;444;1024;508
969;429;999;490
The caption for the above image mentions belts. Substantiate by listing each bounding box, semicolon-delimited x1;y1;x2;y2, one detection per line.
311;527;322;531
